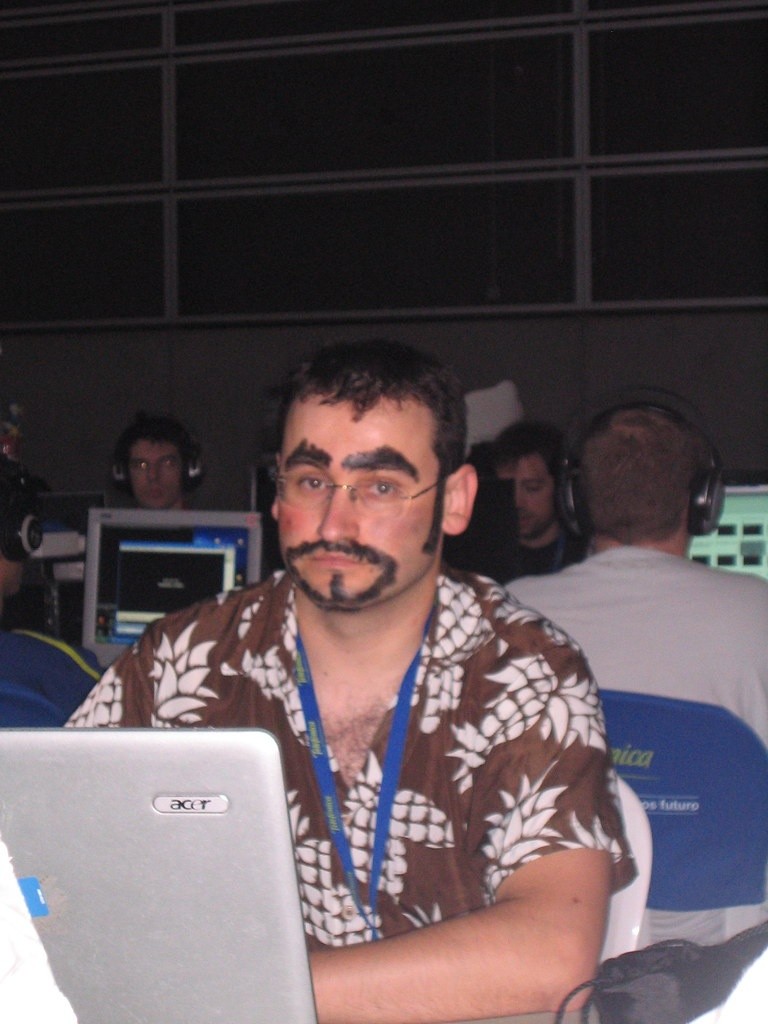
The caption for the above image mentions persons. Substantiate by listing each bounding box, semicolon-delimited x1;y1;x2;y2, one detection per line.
455;399;768;948
112;410;197;512
55;335;622;1024
0;387;33;594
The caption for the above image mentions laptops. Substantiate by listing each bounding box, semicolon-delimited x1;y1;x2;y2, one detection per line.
79;509;262;671
0;728;319;1024
687;485;768;580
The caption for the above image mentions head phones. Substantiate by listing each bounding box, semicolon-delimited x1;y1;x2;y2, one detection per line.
0;454;42;562
110;414;207;494
555;383;725;538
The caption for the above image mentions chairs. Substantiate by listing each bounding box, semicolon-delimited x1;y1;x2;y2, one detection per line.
597;774;653;963
0;631;107;729
594;688;768;912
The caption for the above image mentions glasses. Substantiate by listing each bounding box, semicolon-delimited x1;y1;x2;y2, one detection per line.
270;466;446;519
